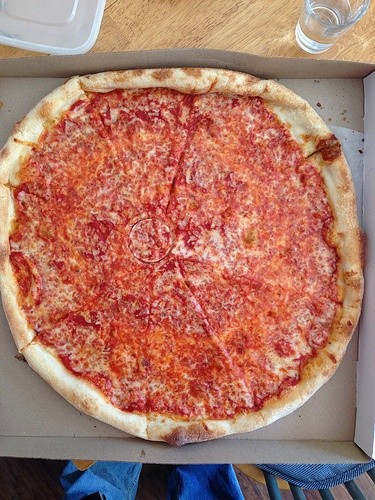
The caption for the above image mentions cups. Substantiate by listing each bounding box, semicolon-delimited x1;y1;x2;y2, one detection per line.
295;1;372;54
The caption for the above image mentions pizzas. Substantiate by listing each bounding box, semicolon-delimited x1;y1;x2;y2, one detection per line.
0;65;366;445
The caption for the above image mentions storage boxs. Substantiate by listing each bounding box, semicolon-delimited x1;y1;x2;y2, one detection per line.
1;47;375;466
